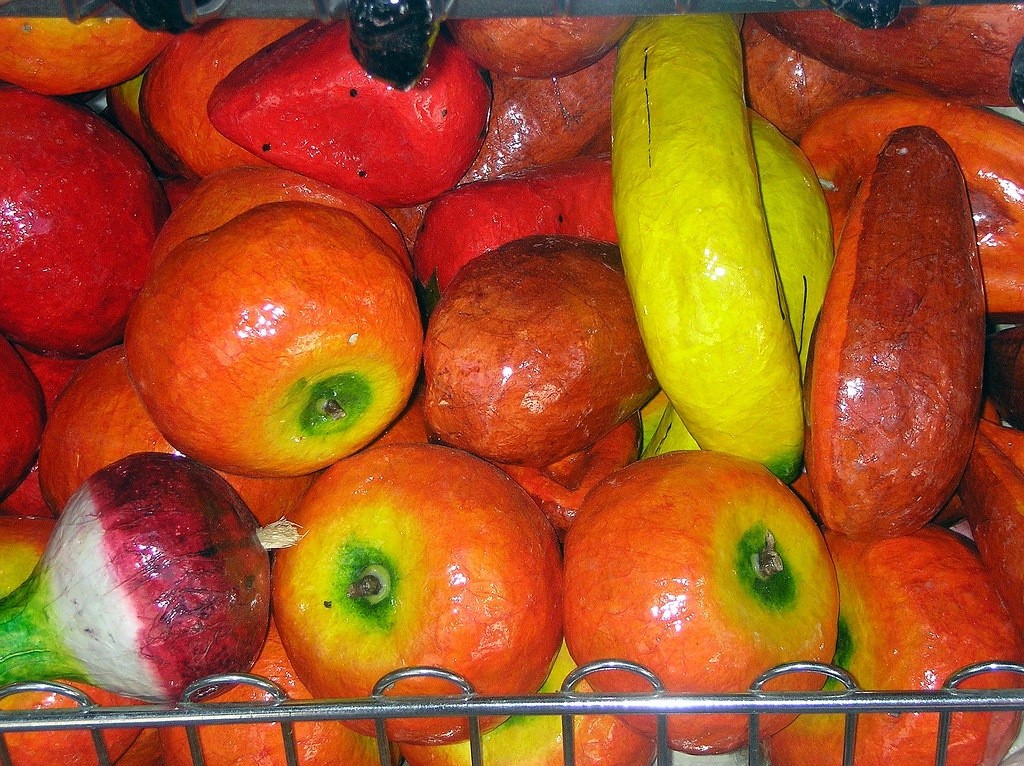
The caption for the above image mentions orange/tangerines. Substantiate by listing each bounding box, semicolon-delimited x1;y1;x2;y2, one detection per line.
0;167;1024;766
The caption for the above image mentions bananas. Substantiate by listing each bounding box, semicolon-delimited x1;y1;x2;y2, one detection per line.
609;14;836;484
